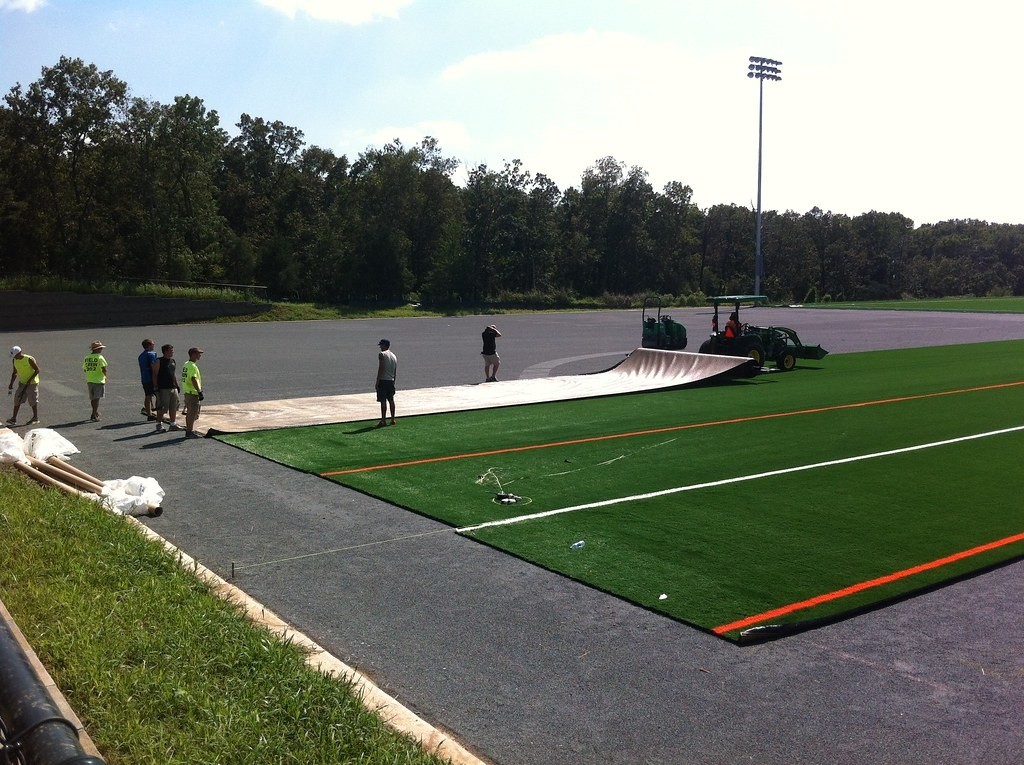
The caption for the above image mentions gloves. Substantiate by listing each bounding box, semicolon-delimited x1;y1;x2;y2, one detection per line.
199;390;204;401
177;386;180;394
153;385;158;391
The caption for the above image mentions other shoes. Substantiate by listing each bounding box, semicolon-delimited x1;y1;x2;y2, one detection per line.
169;424;183;431
375;421;386;427
26;416;40;425
185;431;201;438
391;420;396;425
91;414;101;422
486;378;492;382
489;376;499;382
156;423;166;431
6;418;16;425
147;414;158;421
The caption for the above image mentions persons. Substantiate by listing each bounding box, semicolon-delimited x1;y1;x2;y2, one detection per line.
82;340;109;422
139;339;159;421
152;345;183;431
725;312;739;340
181;348;204;438
481;325;502;382
6;346;40;424
374;339;397;426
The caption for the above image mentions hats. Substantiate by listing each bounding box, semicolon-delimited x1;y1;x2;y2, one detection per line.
491;325;496;328
188;347;203;356
378;339;390;346
9;345;21;360
89;340;106;350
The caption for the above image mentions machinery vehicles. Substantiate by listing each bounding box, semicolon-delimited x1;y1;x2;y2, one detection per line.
698;296;829;378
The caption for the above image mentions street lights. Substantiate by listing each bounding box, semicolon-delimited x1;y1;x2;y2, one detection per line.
747;56;782;298
